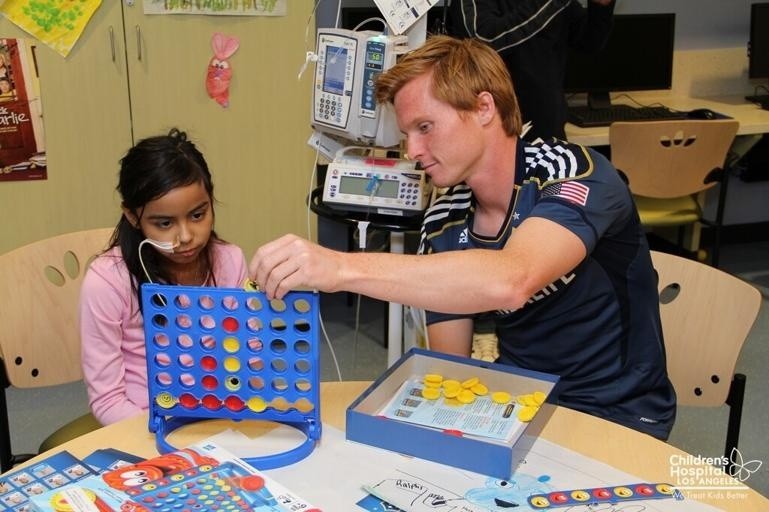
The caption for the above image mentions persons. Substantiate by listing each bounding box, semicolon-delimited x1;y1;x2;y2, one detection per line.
80;130;251;424
251;34;677;441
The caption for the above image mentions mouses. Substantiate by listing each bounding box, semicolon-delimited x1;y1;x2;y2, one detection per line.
688;109;715;120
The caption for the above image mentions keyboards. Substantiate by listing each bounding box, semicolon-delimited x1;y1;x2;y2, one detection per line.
745;95;769;110
569;106;685;127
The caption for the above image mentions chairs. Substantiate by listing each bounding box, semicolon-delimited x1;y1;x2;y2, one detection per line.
649;250;762;477
0;227;117;474
610;120;739;269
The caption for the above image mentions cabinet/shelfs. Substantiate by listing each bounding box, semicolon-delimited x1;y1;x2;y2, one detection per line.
0;0;319;328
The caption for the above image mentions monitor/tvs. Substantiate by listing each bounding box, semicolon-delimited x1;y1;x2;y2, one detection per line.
748;3;769;79
555;12;677;108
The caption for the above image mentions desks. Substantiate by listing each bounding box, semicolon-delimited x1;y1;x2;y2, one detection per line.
1;380;769;512
564;94;769;168
